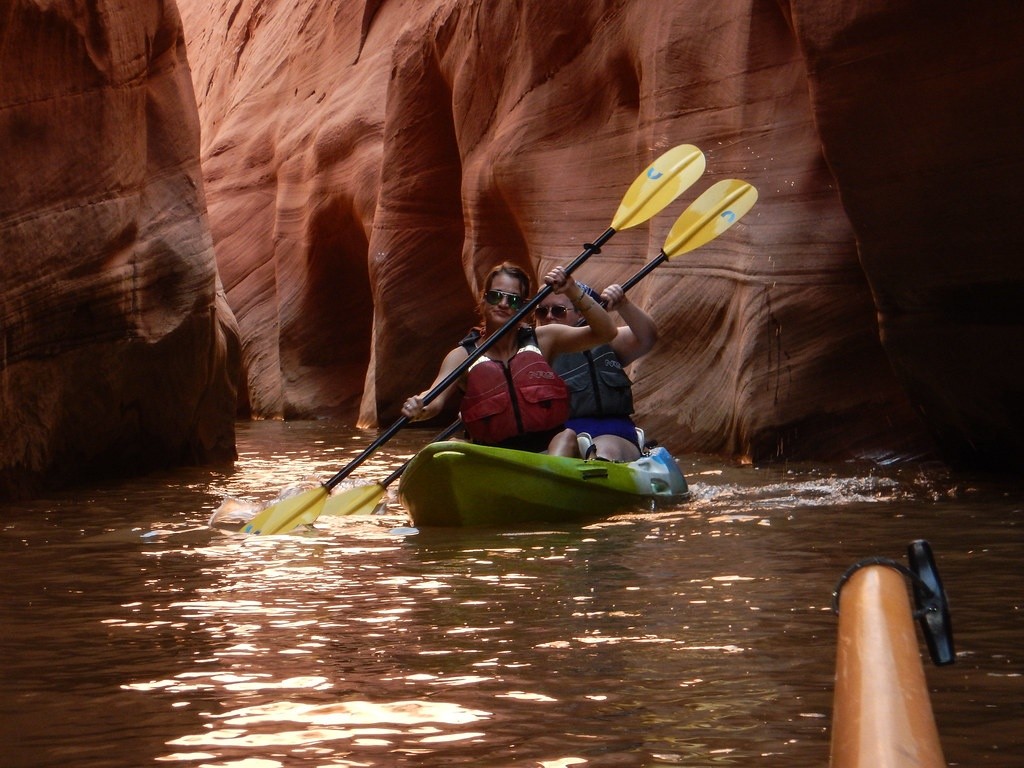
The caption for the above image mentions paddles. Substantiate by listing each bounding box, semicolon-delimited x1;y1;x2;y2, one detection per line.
317;178;759;514
238;144;707;535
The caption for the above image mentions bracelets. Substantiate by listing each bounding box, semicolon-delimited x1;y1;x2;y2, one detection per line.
578;289;587;302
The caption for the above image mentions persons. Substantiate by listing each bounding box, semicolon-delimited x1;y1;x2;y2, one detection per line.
458;284;658;463
401;260;618;457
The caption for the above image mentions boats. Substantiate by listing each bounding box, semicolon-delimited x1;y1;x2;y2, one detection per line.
399;437;692;529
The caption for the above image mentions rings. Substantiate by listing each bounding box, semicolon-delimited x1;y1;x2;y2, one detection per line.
405;404;410;408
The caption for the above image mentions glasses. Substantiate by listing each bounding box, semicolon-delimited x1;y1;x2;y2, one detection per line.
484;289;523;311
534;305;581;320
